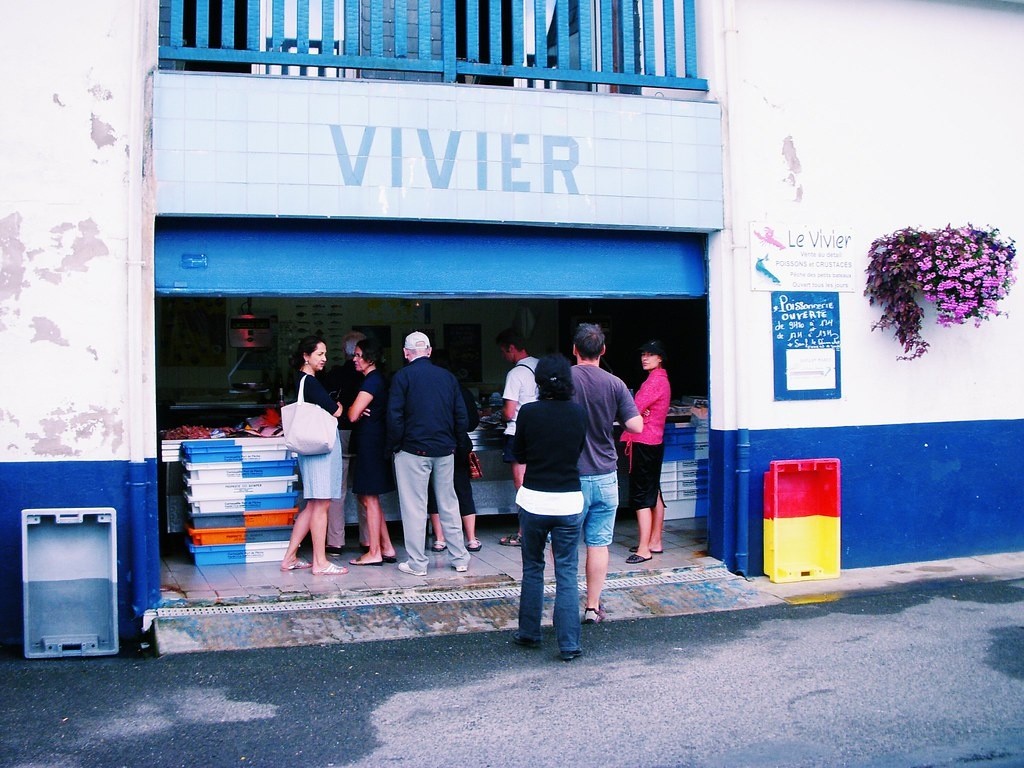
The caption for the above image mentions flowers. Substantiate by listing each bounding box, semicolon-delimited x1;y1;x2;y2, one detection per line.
865;224;1019;360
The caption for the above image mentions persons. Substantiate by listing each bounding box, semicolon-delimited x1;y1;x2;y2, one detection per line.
515;354;589;658
570;323;644;623
387;332;470;575
496;328;540;546
620;341;671;564
280;336;349;575
325;331;370;556
348;339;398;566
427;387;482;552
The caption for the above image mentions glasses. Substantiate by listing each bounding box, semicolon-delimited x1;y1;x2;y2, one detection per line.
353;352;363;359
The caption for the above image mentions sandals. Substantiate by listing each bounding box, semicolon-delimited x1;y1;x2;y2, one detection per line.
467;537;482;551
500;531;522;546
432;541;446;551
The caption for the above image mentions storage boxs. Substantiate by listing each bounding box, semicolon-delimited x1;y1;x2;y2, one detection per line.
182;421;301;566
764;458;842;582
657;419;710;519
22;507;120;657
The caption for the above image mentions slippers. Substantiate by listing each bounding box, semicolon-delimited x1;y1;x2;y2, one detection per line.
348;557;382;566
629;546;663;553
626;554;651;563
312;563;348;575
382;556;396;562
279;558;312;570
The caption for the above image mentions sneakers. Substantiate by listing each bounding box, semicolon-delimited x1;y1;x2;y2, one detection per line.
398;562;427;576
455;565;468;571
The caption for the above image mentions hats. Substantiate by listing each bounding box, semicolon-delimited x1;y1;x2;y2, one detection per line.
639;340;668;361
405;331;430;349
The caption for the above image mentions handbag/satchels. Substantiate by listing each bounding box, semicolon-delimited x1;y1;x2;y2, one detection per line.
281;375;339;455
469;452;484;478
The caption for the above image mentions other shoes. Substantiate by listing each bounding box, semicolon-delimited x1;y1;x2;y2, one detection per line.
585;605;606;623
361;545;370;551
513;631;539;647
330;547;341;556
560;649;581;659
